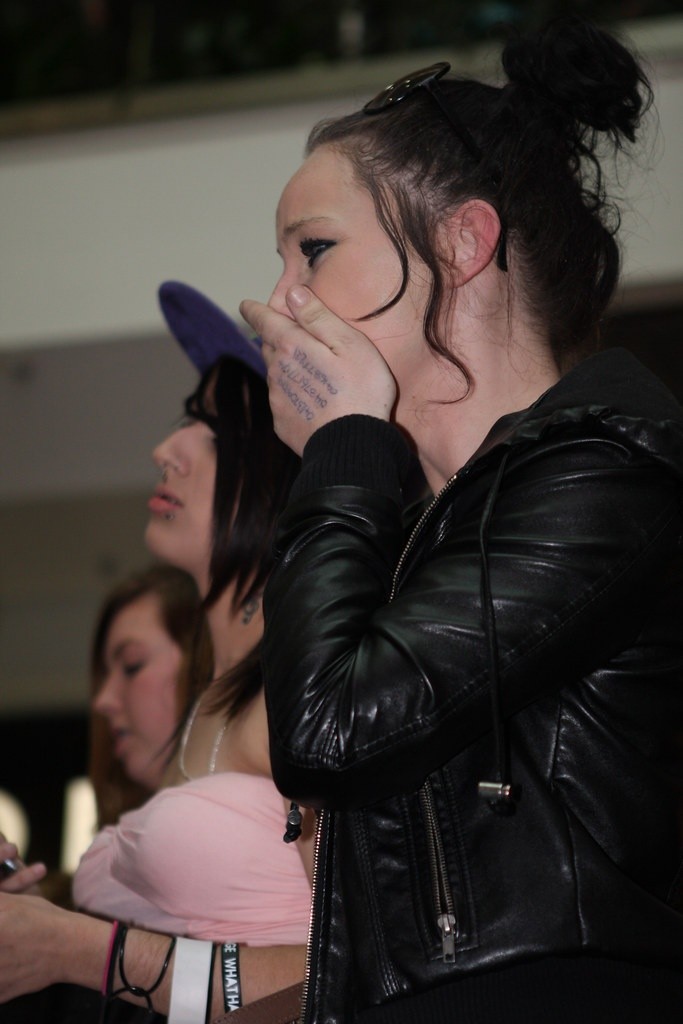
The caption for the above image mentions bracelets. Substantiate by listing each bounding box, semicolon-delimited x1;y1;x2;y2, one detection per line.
220;941;242;1013
103;918;175;1023
166;931;216;1024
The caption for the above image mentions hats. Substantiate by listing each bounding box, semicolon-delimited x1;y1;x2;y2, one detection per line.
157;279;269;380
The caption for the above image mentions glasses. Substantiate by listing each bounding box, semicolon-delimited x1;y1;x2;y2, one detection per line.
362;61;483;159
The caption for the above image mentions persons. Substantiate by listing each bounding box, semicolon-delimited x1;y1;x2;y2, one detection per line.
0;565;215;905
239;19;683;1024
6;278;321;1024
